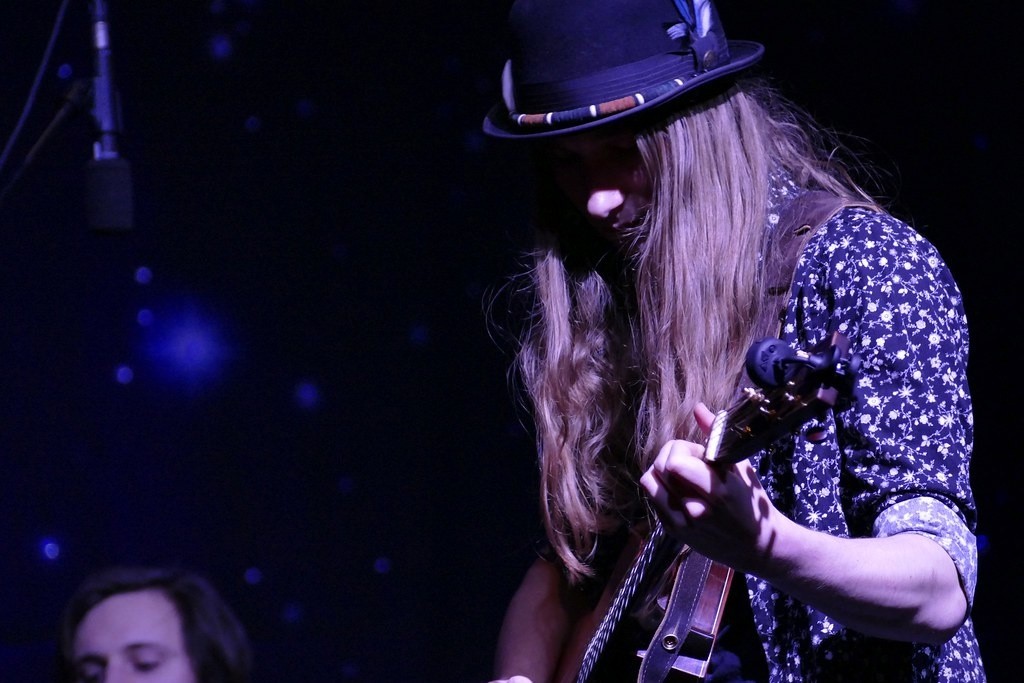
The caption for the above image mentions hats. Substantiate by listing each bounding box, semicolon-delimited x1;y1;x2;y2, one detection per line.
482;0;764;138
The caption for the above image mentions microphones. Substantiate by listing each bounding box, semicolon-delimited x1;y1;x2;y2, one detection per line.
80;15;138;235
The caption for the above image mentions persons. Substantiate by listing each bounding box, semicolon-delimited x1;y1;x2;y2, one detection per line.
59;569;250;683
483;0;987;683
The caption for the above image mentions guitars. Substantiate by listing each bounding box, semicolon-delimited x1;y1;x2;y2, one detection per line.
550;328;862;683
